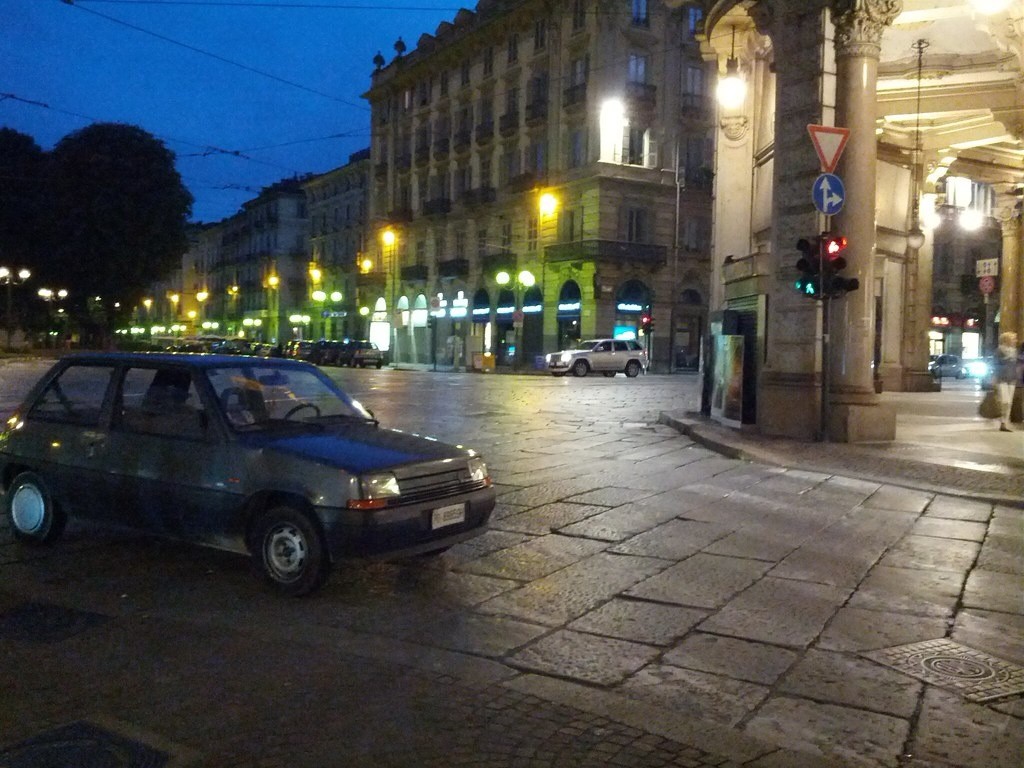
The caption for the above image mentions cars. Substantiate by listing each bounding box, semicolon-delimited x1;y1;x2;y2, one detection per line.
547;339;649;378
0;353;496;596
928;354;995;380
166;338;383;369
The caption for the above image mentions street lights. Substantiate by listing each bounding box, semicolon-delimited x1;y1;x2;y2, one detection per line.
495;271;535;371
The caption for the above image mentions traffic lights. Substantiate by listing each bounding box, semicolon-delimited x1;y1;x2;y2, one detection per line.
796;235;846;298
641;304;655;334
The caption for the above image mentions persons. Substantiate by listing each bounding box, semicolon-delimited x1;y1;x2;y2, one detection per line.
992;332;1024;433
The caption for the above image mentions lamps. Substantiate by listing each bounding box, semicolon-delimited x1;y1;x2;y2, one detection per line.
906;40;929;250
716;25;748;109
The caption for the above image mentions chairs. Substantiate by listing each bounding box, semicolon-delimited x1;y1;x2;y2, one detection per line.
218;387;255;427
140;386;200;436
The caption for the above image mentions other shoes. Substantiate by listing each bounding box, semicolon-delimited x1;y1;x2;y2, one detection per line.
999;427;1013;432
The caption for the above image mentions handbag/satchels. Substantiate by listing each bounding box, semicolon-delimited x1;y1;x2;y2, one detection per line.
979;388;1002;419
